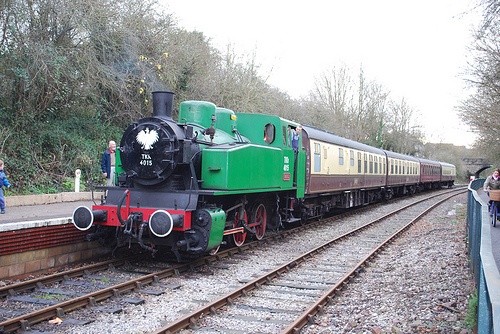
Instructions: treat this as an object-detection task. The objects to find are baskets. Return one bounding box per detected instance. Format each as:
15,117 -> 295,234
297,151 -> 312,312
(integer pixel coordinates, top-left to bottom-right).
489,190 -> 500,201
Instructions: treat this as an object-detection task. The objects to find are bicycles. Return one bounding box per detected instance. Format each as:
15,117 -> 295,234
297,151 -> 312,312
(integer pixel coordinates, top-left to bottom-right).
485,188 -> 500,227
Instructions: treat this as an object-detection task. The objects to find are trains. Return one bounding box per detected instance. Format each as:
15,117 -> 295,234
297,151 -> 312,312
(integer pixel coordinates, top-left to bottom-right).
73,90 -> 457,264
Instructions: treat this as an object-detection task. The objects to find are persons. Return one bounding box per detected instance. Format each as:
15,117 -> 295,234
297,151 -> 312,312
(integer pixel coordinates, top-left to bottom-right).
0,160 -> 11,214
101,140 -> 117,196
484,168 -> 500,221
